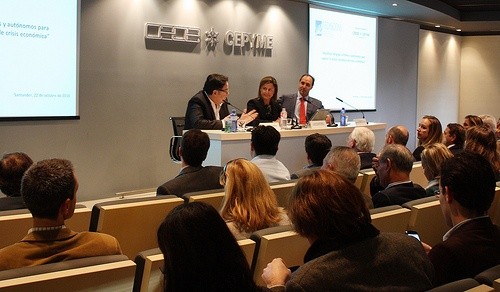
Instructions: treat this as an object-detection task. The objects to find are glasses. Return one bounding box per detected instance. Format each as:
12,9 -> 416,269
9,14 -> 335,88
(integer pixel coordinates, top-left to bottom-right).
221,88 -> 229,92
434,188 -> 444,199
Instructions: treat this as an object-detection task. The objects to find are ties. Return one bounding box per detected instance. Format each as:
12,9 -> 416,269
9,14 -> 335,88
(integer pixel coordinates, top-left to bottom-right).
300,98 -> 306,124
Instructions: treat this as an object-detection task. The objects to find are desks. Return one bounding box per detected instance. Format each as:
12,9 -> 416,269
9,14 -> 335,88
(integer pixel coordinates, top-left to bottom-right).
199,121 -> 387,175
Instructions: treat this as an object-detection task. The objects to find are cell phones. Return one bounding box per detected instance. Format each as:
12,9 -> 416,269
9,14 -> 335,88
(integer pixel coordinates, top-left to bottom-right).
406,230 -> 420,243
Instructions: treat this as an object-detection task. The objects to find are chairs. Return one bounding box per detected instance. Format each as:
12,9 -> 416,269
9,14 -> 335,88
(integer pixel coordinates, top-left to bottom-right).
169,116 -> 185,163
0,161 -> 500,292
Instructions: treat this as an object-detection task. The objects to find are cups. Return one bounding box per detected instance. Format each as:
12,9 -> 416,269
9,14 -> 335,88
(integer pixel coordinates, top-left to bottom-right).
237,120 -> 245,130
287,118 -> 292,130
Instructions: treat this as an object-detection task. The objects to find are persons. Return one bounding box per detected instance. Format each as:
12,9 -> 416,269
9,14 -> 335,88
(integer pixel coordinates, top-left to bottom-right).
250,125 -> 290,185
0,152 -> 34,197
292,114 -> 500,209
184,74 -> 258,130
0,159 -> 122,271
421,151 -> 500,288
262,169 -> 436,292
156,128 -> 224,198
156,202 -> 271,292
219,158 -> 290,241
247,76 -> 284,126
278,74 -> 334,124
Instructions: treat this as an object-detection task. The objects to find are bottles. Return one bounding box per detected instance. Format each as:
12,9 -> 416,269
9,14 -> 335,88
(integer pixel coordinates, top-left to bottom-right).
340,107 -> 347,126
280,107 -> 287,129
229,109 -> 238,131
326,115 -> 331,125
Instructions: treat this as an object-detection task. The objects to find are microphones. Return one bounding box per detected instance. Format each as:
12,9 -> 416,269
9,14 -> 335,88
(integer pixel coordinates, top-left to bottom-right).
274,101 -> 301,128
305,99 -> 330,125
222,99 -> 257,128
335,97 -> 366,123
